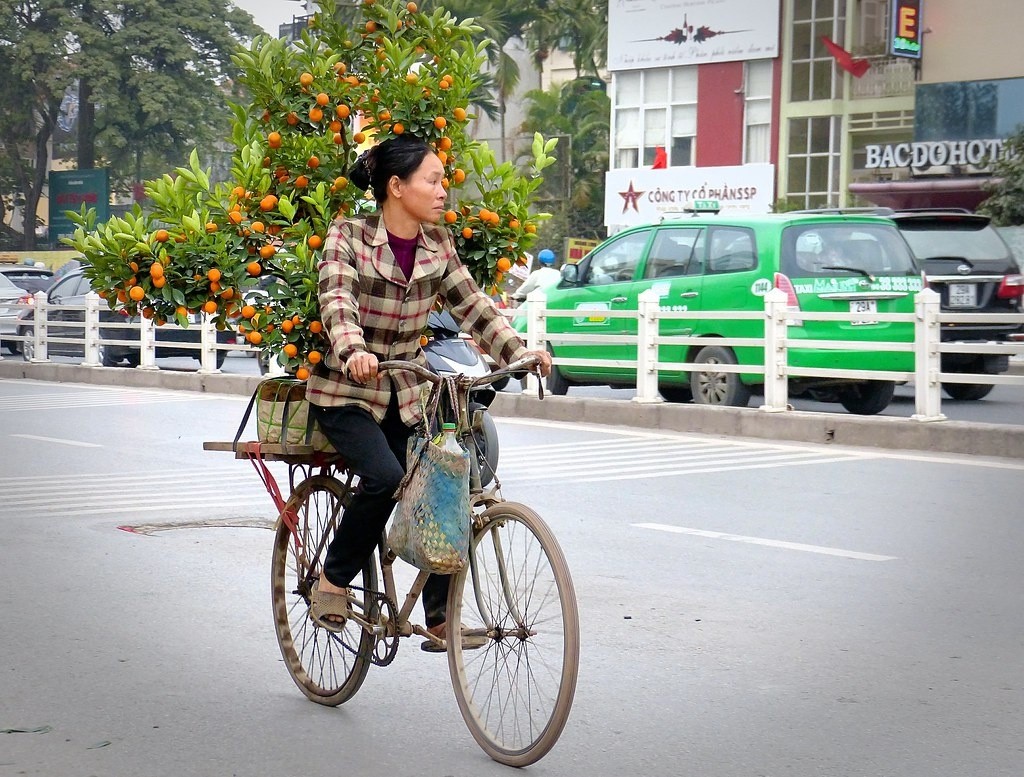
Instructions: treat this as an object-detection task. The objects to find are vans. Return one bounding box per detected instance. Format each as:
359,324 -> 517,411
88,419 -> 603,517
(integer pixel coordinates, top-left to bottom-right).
510,213 -> 927,416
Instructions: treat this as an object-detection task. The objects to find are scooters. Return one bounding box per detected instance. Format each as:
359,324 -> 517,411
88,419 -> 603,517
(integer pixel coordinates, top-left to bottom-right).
421,311 -> 500,490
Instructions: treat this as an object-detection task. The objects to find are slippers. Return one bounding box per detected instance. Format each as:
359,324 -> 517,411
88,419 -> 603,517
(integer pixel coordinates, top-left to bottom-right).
421,622 -> 489,652
312,580 -> 348,633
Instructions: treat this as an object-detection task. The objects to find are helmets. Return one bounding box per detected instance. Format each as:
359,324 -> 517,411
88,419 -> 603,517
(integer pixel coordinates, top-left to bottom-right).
538,250 -> 555,266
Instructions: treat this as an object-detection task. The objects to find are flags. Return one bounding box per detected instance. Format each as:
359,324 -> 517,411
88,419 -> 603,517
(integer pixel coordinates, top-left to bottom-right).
821,36 -> 872,78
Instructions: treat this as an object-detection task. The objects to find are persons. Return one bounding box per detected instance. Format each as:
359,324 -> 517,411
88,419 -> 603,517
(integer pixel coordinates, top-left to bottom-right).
646,238 -> 677,278
24,258 -> 46,269
306,134 -> 552,651
512,249 -> 562,322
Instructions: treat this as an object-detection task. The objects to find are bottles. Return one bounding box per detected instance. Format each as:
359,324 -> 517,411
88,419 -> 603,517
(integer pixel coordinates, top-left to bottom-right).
437,423 -> 465,459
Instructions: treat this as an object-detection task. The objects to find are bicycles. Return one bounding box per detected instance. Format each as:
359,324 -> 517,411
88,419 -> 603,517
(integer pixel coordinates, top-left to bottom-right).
271,356 -> 582,768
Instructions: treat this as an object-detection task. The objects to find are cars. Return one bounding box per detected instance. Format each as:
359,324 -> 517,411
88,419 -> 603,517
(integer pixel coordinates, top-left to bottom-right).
17,266 -> 235,371
0,262 -> 54,356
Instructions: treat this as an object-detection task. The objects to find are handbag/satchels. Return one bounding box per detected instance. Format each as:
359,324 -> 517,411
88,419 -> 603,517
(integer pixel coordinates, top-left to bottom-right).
386,374 -> 470,575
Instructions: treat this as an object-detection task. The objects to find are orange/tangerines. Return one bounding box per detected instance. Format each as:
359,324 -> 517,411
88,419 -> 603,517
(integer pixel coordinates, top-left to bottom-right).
93,0 -> 536,380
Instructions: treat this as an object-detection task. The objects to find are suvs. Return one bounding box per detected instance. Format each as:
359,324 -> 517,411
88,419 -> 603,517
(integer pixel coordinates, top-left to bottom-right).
732,206 -> 1024,400
